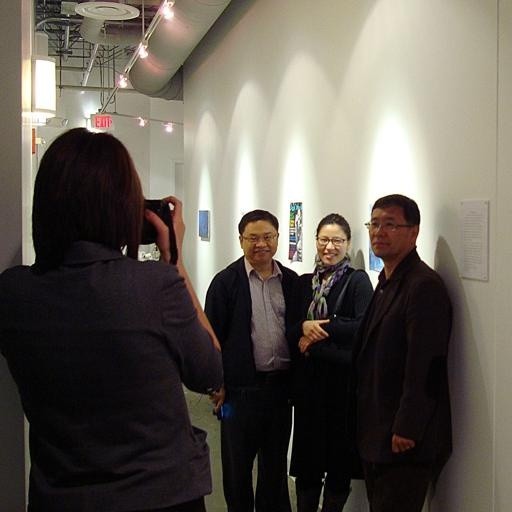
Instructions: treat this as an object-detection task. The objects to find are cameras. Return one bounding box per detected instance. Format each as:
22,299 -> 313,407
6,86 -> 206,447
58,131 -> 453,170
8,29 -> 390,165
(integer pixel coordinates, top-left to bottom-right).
140,199 -> 170,245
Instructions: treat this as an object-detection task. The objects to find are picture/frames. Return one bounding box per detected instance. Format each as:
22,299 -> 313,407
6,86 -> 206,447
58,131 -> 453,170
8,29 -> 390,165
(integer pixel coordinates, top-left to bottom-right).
198,210 -> 211,239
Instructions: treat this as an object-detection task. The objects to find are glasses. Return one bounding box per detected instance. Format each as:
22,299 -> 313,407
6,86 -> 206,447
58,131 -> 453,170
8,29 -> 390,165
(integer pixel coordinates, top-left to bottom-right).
244,234 -> 276,242
317,237 -> 346,246
365,222 -> 410,232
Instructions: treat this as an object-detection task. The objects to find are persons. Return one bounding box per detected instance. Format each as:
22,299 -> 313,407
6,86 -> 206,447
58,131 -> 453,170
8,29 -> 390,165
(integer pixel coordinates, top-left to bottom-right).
1,127 -> 222,511
202,210 -> 301,511
286,212 -> 375,512
347,194 -> 454,512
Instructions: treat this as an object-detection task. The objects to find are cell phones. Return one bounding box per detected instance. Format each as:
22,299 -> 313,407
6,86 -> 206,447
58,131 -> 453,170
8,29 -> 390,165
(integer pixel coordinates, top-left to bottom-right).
217,405 -> 223,421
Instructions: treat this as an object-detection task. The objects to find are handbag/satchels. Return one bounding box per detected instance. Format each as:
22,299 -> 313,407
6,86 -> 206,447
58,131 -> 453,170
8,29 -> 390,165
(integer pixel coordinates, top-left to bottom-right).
307,314 -> 357,359
